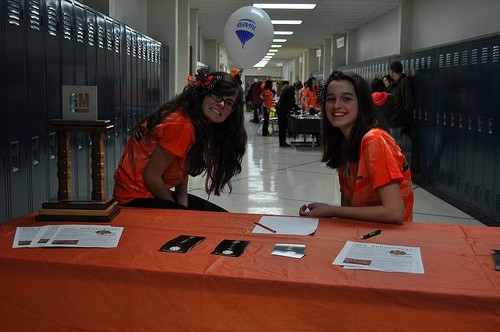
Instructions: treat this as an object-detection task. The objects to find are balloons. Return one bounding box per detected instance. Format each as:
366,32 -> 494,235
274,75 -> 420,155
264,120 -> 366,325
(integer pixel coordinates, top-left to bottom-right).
223,6 -> 274,70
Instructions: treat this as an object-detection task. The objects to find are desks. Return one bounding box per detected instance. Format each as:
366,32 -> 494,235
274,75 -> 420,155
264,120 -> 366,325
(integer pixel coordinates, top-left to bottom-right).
0,206 -> 499,332
291,113 -> 322,150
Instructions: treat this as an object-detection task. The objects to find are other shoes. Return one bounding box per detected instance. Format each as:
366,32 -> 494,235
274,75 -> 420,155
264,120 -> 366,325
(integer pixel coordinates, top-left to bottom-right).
280,143 -> 292,147
264,134 -> 272,136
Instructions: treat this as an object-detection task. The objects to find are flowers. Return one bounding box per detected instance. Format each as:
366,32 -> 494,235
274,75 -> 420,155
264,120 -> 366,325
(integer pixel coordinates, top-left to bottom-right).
187,66 -> 220,90
231,69 -> 243,86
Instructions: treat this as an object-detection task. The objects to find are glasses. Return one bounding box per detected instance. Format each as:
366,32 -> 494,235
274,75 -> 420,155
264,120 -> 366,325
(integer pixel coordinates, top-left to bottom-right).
209,91 -> 236,111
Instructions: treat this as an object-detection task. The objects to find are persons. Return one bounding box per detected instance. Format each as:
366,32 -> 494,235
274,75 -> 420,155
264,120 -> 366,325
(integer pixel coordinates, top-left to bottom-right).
371,61 -> 413,167
113,66 -> 247,213
275,80 -> 304,147
294,77 -> 326,115
298,70 -> 414,225
244,79 -> 289,136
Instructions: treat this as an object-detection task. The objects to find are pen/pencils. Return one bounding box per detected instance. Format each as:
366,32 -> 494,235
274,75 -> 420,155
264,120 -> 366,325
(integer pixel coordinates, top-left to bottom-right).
254,222 -> 276,233
362,230 -> 381,239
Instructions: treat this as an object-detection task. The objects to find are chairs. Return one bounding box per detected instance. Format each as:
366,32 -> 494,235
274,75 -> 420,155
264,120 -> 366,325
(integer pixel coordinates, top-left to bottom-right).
272,118 -> 280,133
256,109 -> 273,135
